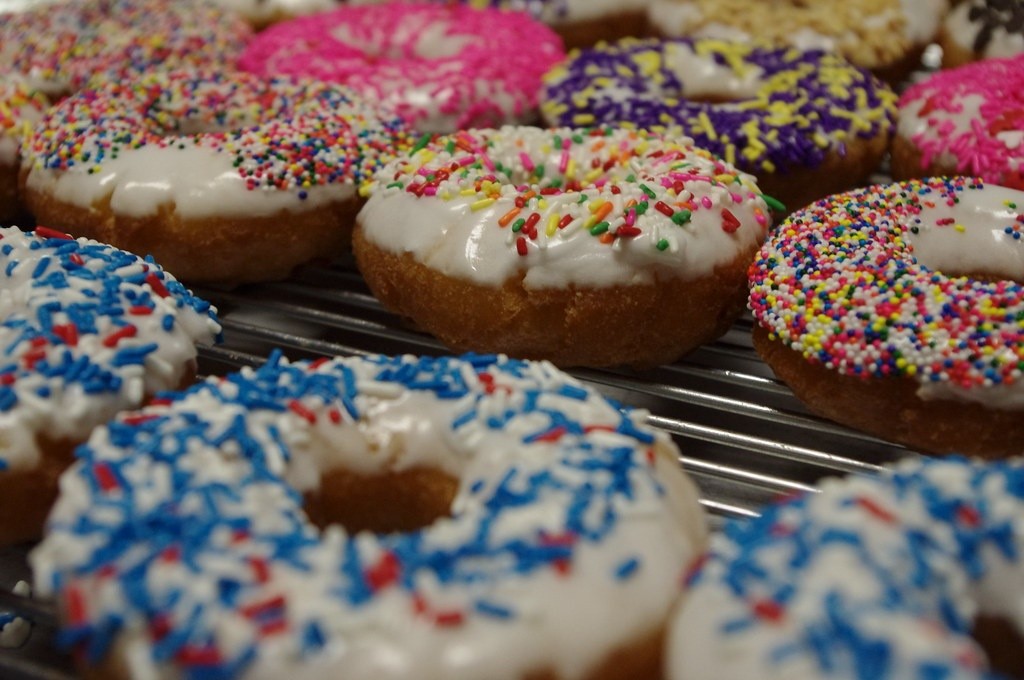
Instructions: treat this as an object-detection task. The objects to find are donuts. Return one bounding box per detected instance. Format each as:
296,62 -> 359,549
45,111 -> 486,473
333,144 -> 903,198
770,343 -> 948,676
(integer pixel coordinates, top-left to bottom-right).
0,0 -> 1024,680
